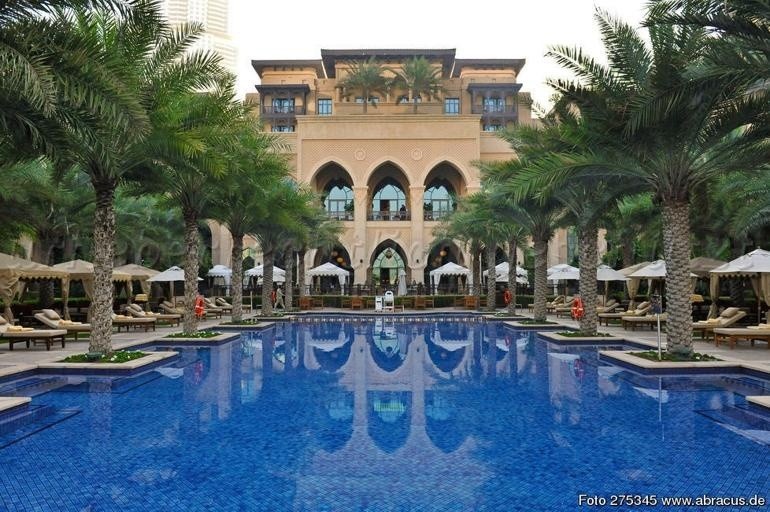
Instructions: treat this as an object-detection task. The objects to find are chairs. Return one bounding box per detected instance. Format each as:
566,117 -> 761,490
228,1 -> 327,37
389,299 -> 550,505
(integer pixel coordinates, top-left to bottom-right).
295,294 -> 489,311
527,294 -> 770,352
0,296 -> 254,352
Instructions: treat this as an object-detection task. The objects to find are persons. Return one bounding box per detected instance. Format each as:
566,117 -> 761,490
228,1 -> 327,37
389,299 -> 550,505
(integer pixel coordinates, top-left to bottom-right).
273,285 -> 286,310
423,202 -> 434,221
400,203 -> 407,220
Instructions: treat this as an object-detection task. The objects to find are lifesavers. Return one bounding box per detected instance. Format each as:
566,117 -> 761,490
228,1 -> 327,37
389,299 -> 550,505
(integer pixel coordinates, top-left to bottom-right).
504,291 -> 510,303
572,298 -> 583,318
272,291 -> 276,301
195,297 -> 205,314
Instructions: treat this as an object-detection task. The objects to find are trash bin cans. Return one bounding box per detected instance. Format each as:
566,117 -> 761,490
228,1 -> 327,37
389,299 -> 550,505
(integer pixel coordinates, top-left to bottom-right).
375,296 -> 382,312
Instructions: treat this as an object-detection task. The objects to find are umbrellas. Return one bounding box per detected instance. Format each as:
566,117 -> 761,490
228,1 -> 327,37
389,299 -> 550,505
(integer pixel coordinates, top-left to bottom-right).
716,420 -> 770,448
545,263 -> 576,275
708,245 -> 770,325
145,265 -> 205,309
397,323 -> 409,361
398,269 -> 408,297
628,259 -> 699,313
596,264 -> 632,307
548,267 -> 579,302
546,350 -> 671,403
154,364 -> 185,379
